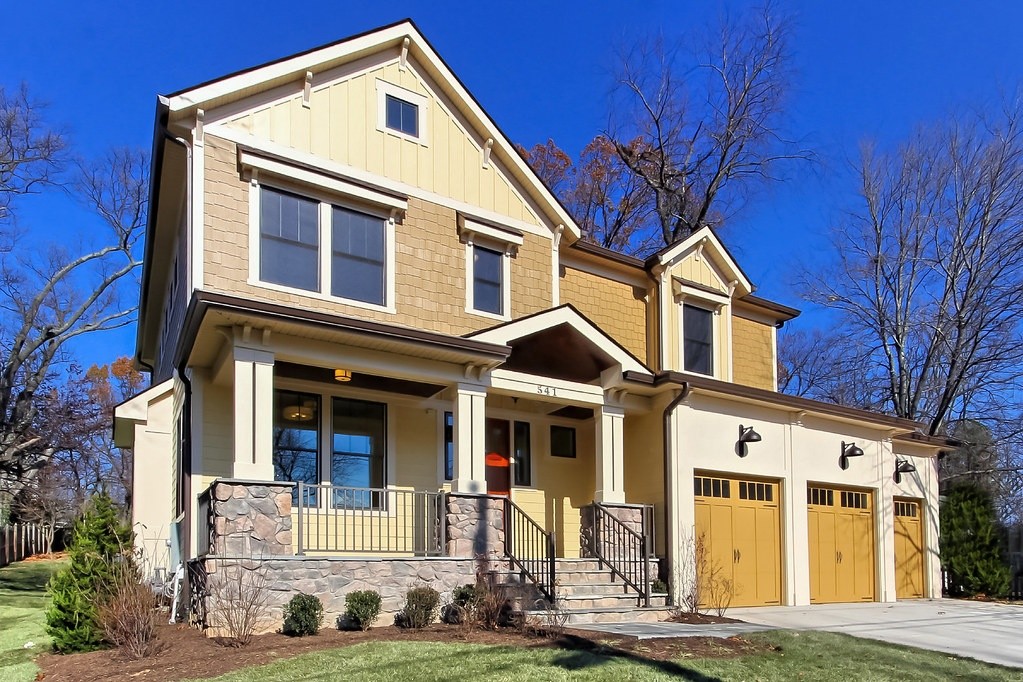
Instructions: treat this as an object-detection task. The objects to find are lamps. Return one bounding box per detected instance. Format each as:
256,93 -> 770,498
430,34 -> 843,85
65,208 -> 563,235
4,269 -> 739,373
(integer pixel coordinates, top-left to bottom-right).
895,457 -> 915,482
841,439 -> 864,466
334,369 -> 352,382
739,423 -> 764,455
283,405 -> 314,422
